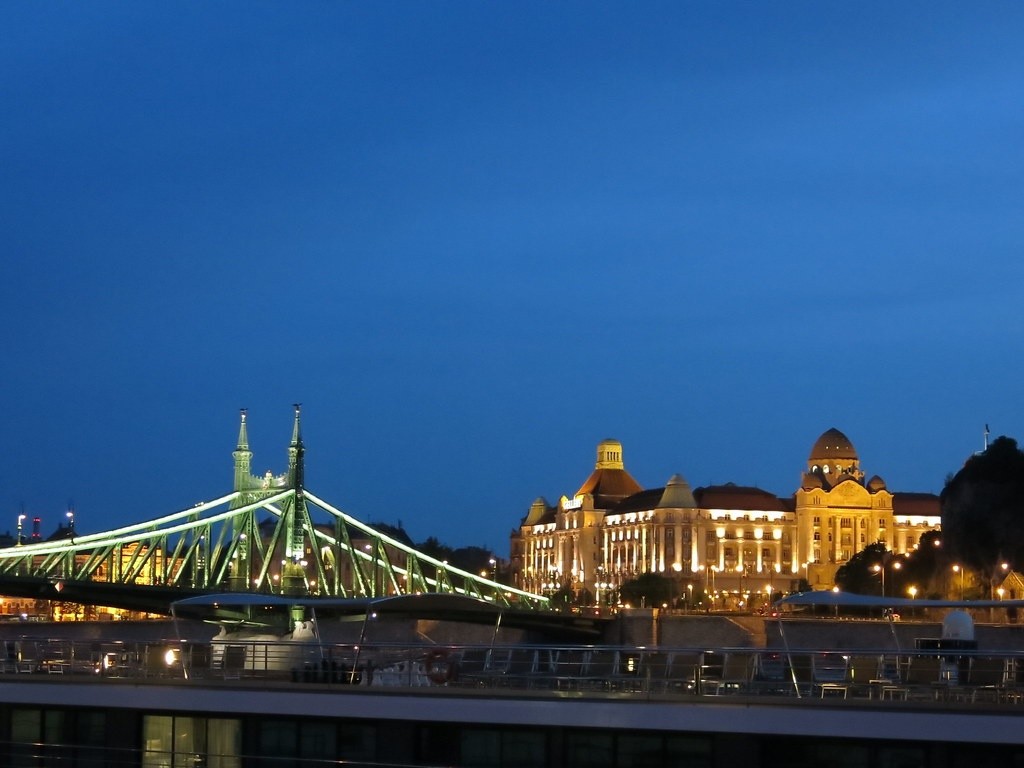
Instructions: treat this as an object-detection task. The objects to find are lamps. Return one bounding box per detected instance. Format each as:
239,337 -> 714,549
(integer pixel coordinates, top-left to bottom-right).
297,558 -> 309,567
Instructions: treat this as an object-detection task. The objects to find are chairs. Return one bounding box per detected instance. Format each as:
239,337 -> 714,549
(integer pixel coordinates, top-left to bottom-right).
0,638 -> 1023,708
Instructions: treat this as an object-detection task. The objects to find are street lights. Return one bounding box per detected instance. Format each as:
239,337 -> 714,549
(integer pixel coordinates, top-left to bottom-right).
736,566 -> 743,611
908,586 -> 917,620
833,585 -> 839,618
490,560 -> 497,583
874,565 -> 884,596
952,565 -> 964,601
